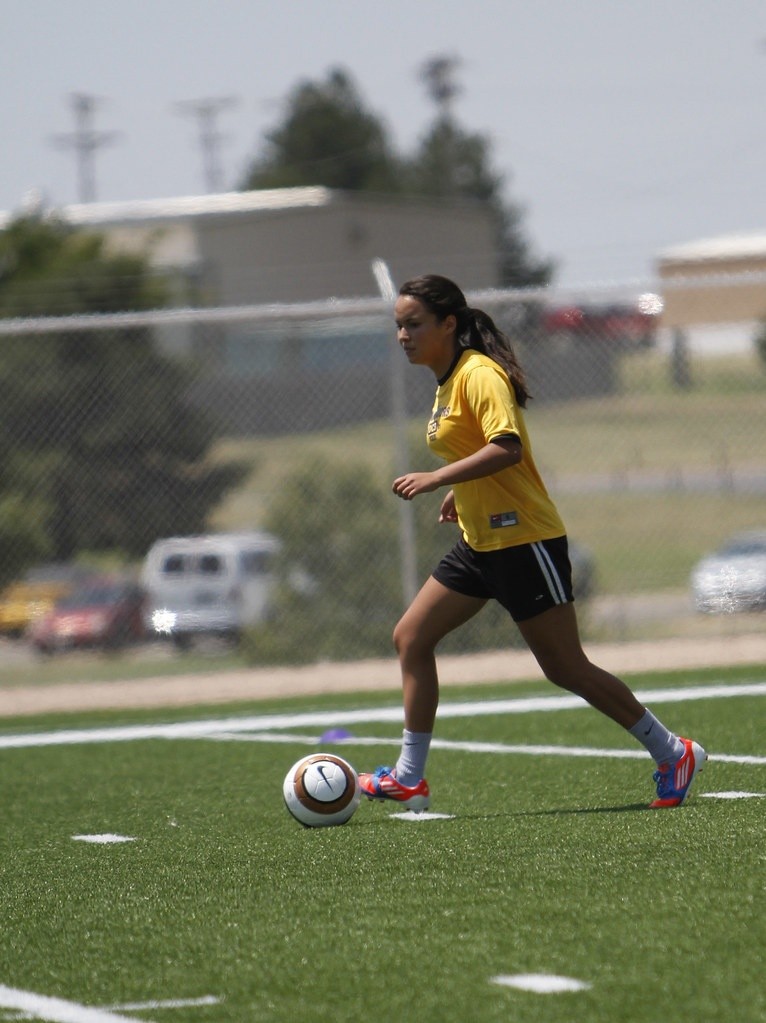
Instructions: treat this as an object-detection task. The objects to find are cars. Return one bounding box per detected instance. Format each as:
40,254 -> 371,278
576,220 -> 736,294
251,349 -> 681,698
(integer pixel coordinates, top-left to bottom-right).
0,558 -> 155,654
686,531 -> 766,612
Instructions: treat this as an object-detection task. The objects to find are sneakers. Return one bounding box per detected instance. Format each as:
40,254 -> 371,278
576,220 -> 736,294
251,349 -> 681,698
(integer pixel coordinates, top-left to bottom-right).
359,767 -> 430,815
651,738 -> 707,809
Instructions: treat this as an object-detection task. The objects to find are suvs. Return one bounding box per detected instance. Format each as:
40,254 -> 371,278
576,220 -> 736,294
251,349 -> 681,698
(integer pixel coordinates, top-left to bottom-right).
137,528 -> 316,649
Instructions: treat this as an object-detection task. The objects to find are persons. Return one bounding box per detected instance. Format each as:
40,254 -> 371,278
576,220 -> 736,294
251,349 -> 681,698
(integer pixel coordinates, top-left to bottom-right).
357,272 -> 708,813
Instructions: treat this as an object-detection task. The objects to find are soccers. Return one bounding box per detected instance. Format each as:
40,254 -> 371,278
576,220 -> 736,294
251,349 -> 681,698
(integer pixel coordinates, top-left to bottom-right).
280,752 -> 362,829
